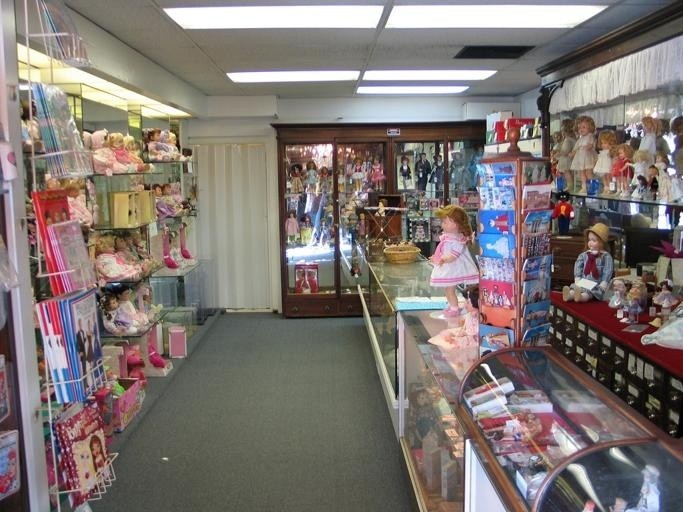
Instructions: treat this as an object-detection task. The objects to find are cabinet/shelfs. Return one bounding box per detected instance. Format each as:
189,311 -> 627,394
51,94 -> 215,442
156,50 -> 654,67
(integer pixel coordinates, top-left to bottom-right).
56,81 -> 167,339
358,248 -> 468,442
128,105 -> 201,378
455,346 -> 683,511
536,2 -> 683,442
399,309 -> 478,512
270,122 -> 391,318
392,122 -> 486,260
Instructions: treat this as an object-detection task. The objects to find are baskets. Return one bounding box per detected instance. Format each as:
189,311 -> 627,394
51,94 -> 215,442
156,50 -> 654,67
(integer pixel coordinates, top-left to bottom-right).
383,246 -> 421,264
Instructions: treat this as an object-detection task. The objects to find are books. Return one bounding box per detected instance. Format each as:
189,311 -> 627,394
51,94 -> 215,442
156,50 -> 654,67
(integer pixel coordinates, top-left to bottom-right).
29,83 -> 92,180
35,291 -> 108,402
55,396 -> 112,506
29,190 -> 94,296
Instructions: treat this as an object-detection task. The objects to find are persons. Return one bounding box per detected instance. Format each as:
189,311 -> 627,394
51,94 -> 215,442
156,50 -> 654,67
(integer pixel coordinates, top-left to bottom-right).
81,130 -> 164,335
562,223 -> 678,324
145,129 -> 191,268
428,206 -> 479,315
550,116 -> 683,201
399,154 -> 443,190
286,156 -> 384,245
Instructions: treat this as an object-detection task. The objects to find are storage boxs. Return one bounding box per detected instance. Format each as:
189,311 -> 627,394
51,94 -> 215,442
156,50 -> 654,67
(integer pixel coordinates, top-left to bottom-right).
108,377 -> 140,433
